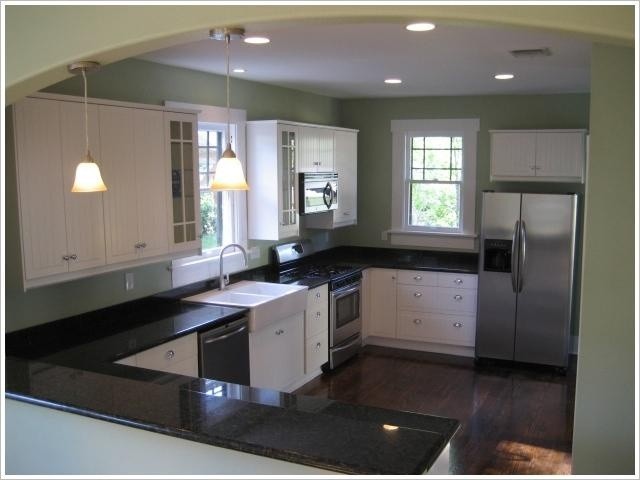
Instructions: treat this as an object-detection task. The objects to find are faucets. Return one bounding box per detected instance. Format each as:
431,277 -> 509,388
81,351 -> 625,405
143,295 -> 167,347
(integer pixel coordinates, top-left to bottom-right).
218,243 -> 248,290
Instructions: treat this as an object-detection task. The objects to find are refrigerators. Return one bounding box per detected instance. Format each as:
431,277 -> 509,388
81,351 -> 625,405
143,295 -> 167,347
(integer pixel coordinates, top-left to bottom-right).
474,188 -> 578,378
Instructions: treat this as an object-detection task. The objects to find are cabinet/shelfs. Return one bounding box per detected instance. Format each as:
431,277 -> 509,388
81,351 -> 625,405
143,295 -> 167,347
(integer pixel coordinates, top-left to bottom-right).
363,267 -> 398,346
305,284 -> 330,375
489,129 -> 587,183
297,122 -> 337,173
244,119 -> 299,241
168,110 -> 201,259
398,270 -> 479,357
114,332 -> 198,380
6,89 -> 105,292
249,310 -> 305,393
104,96 -> 173,272
334,127 -> 360,229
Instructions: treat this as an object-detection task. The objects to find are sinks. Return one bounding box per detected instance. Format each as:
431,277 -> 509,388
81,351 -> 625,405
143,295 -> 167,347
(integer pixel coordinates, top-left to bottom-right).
180,290 -> 275,309
226,279 -> 307,297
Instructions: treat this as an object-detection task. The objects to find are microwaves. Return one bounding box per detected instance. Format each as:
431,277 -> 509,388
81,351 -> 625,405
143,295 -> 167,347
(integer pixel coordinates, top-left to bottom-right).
298,171 -> 339,217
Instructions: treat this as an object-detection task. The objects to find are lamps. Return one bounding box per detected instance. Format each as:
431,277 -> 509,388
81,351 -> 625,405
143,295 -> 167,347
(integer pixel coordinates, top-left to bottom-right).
207,27 -> 250,191
68,61 -> 107,193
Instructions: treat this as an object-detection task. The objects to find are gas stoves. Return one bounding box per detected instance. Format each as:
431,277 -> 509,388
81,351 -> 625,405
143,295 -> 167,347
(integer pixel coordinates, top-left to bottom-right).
270,254 -> 369,290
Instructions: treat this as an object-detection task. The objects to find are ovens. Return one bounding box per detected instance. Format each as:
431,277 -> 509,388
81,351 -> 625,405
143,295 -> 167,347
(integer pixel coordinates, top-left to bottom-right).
328,281 -> 364,371
